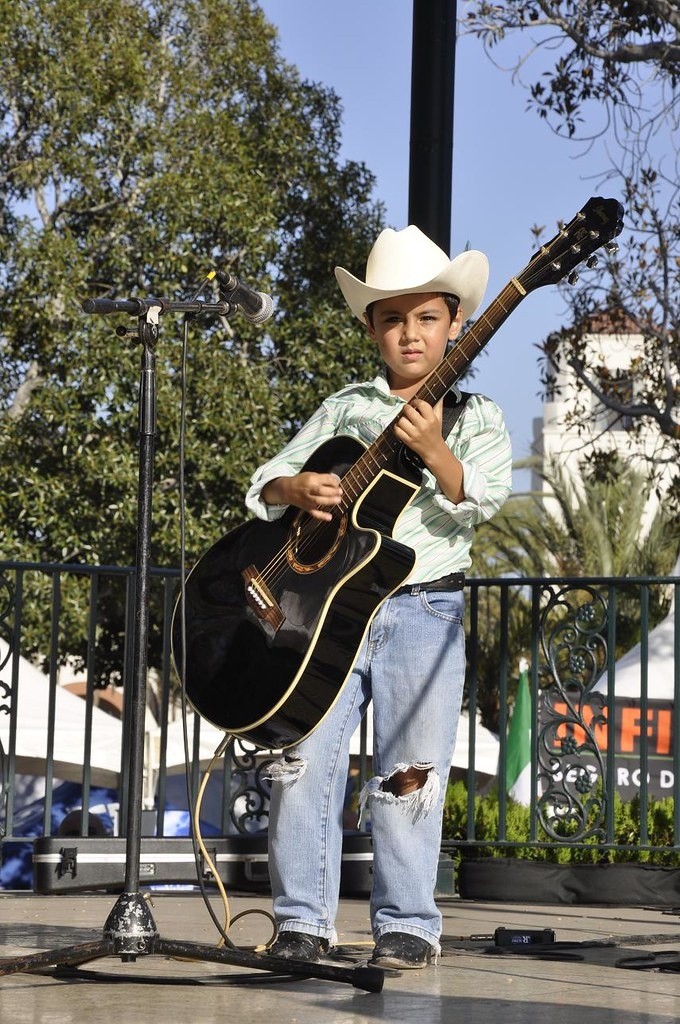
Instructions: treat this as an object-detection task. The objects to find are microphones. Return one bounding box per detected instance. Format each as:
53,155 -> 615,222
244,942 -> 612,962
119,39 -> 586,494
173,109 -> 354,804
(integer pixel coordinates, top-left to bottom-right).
211,266 -> 273,323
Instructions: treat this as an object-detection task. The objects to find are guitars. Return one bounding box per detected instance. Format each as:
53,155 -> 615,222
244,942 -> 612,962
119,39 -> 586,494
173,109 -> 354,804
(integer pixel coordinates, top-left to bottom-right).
167,197 -> 624,749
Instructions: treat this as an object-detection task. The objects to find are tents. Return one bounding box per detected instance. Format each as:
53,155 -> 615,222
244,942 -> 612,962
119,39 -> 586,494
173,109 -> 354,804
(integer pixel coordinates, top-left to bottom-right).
0,638 -> 541,834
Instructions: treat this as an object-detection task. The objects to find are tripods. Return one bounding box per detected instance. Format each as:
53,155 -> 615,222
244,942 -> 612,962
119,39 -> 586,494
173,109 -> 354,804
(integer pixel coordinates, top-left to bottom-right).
0,299 -> 385,993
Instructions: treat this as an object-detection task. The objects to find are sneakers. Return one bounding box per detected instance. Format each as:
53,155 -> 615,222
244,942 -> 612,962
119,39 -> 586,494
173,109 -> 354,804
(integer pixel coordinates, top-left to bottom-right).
269,931 -> 329,964
373,931 -> 432,968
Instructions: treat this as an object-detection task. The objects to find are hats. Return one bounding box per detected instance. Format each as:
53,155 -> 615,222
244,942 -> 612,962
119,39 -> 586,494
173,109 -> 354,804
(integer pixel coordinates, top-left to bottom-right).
335,225 -> 489,323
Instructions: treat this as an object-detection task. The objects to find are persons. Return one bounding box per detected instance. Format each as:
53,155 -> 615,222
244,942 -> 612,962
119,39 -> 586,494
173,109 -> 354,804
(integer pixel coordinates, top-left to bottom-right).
244,225 -> 511,966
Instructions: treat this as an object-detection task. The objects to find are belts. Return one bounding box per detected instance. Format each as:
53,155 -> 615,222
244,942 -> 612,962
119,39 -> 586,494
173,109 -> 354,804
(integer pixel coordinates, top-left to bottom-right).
386,572 -> 466,599
